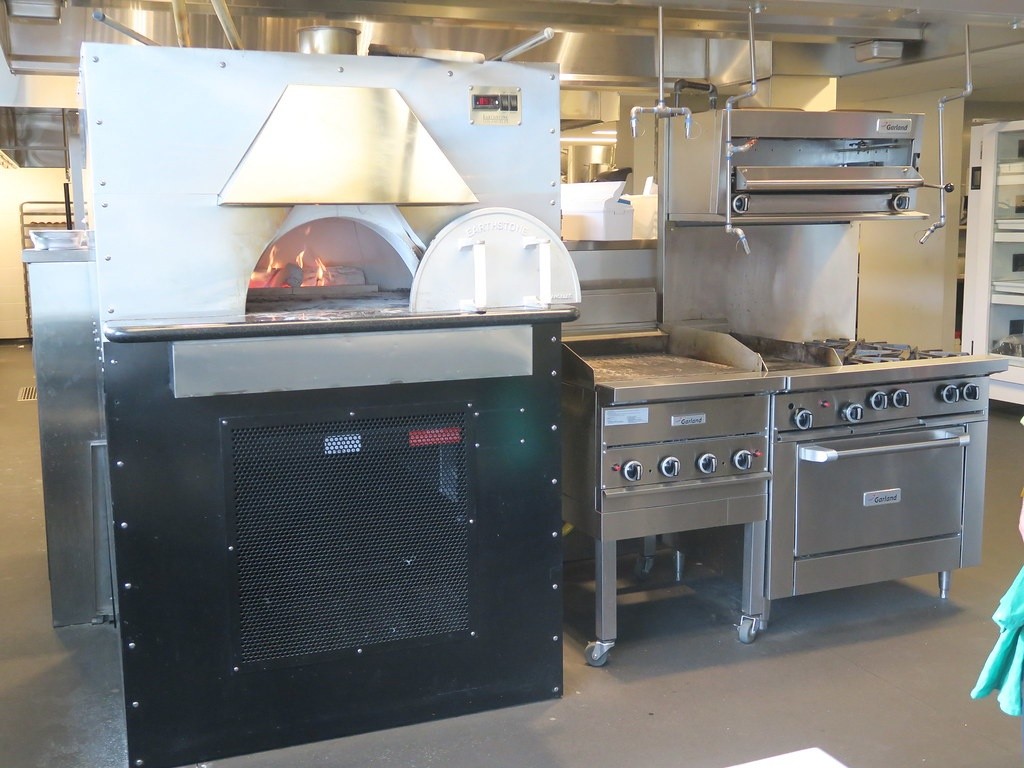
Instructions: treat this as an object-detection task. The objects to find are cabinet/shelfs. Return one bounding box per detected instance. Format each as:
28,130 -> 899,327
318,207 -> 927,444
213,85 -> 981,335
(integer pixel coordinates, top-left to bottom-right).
961,120 -> 1024,405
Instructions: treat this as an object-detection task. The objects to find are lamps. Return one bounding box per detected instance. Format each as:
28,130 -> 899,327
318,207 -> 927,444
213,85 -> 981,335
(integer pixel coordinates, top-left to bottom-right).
854,40 -> 904,64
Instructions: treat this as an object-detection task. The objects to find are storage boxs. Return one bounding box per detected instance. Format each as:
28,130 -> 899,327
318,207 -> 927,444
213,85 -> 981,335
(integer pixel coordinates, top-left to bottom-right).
561,181 -> 634,241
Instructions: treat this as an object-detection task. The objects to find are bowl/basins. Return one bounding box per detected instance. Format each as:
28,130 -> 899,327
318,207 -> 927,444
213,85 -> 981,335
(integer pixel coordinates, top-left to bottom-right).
27,230 -> 87,251
